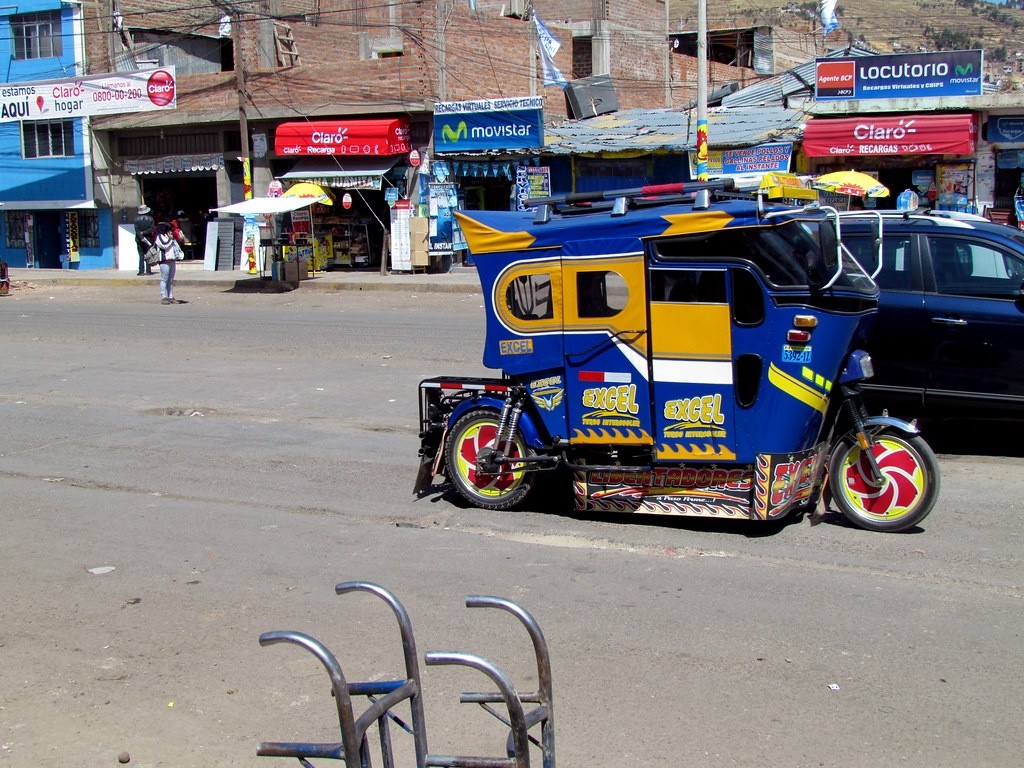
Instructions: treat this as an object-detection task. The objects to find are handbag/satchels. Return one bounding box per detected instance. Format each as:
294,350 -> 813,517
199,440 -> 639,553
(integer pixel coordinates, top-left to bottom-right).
178,230 -> 185,242
144,247 -> 158,265
171,240 -> 184,260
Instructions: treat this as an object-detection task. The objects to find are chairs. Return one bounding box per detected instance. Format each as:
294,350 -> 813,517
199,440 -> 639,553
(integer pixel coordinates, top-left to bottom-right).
932,241 -> 985,295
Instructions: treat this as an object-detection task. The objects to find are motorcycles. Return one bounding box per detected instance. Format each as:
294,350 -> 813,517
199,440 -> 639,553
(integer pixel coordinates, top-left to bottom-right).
412,171 -> 942,532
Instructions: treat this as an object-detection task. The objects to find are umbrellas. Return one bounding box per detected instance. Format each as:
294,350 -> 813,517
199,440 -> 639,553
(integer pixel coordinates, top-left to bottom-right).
812,168 -> 890,211
280,183 -> 336,271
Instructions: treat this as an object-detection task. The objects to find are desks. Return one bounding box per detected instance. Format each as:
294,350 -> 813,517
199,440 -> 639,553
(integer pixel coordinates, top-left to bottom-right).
260,244 -> 315,280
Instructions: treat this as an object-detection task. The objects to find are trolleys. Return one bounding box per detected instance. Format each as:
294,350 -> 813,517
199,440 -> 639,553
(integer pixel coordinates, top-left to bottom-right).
422,593 -> 556,767
258,581 -> 429,768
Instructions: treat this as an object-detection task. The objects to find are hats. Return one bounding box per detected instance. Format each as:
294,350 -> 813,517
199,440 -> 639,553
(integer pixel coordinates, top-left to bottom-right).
138,204 -> 151,214
178,211 -> 184,215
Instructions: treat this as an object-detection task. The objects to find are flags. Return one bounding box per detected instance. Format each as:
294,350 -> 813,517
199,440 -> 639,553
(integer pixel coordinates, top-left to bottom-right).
533,15 -> 565,85
818,0 -> 839,36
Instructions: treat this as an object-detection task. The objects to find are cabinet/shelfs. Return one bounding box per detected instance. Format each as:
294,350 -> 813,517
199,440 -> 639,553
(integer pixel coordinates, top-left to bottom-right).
281,223 -> 373,272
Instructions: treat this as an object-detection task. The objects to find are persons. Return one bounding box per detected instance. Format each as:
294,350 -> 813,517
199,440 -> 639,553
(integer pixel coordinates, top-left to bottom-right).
696,271 -> 752,319
178,211 -> 191,242
135,204 -> 155,276
851,200 -> 865,210
155,222 -> 179,304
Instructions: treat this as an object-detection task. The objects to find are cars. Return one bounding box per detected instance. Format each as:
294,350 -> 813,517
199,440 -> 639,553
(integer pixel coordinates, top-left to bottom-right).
830,208 -> 1023,457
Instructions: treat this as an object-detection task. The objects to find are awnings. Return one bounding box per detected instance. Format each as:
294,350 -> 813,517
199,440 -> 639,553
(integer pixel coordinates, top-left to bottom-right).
274,118 -> 412,156
803,112 -> 976,157
209,195 -> 321,279
274,156 -> 401,189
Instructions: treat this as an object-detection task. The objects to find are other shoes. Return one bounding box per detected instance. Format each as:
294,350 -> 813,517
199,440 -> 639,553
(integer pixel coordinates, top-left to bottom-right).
161,297 -> 175,304
147,272 -> 153,275
137,272 -> 144,276
